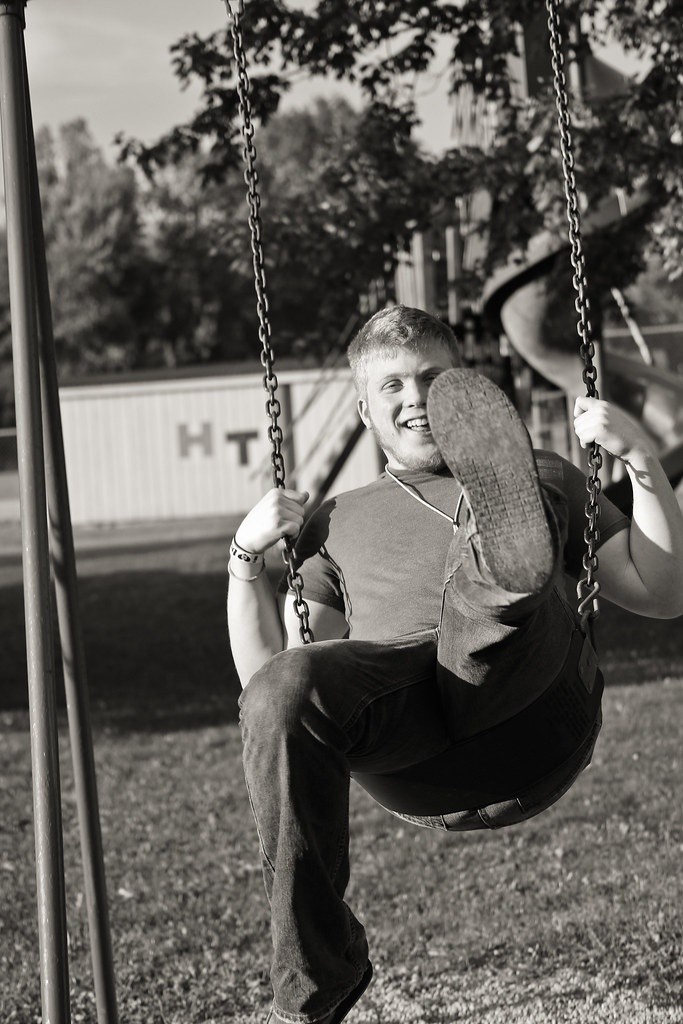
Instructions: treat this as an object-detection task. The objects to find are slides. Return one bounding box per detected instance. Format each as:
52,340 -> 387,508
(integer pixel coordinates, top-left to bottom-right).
474,172 -> 683,519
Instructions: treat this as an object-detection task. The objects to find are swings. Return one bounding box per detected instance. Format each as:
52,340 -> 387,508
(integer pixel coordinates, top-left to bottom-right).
214,0 -> 614,839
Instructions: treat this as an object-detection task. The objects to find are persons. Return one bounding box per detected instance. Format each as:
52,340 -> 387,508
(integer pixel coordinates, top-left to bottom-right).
227,305 -> 683,1020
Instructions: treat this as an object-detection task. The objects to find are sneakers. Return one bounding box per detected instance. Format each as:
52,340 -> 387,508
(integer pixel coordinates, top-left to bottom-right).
263,963 -> 375,1024
424,366 -> 556,593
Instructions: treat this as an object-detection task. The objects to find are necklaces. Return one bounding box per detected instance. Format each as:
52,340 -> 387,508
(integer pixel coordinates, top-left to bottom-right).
384,461 -> 464,536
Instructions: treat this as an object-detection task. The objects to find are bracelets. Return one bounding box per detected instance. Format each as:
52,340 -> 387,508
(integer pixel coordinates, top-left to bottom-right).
229,535 -> 264,565
228,558 -> 266,582
